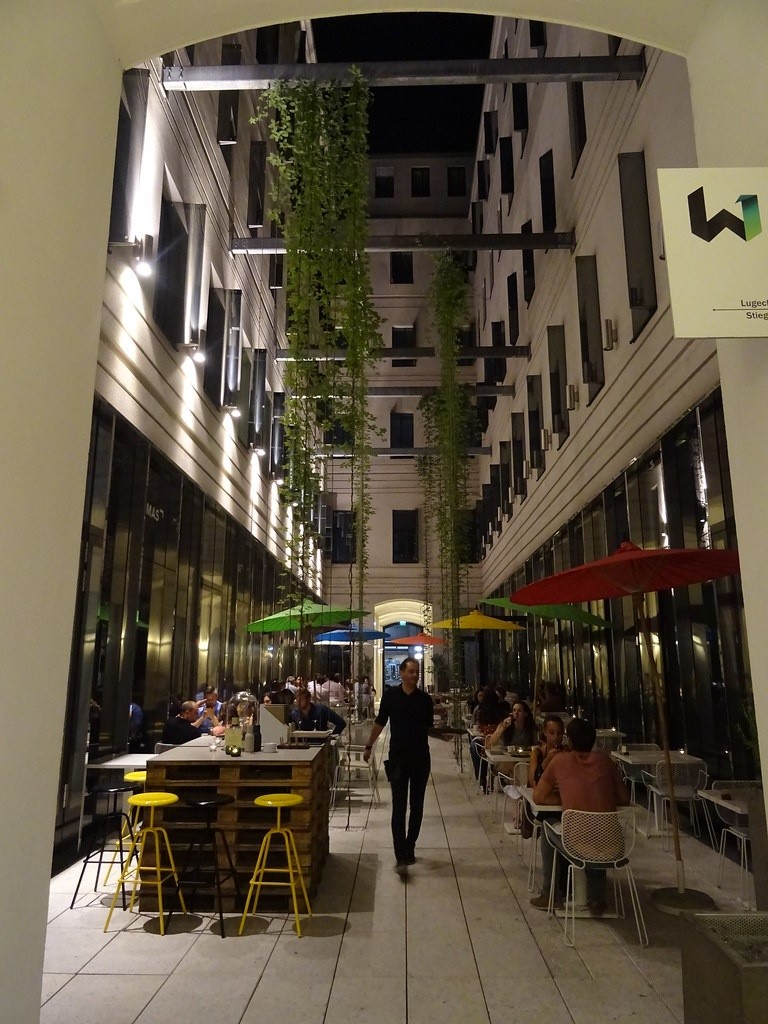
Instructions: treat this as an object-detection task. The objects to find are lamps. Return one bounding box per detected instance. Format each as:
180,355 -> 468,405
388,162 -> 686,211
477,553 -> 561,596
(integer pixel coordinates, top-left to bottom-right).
523,460 -> 532,479
132,234 -> 154,277
498,507 -> 505,521
602,319 -> 618,351
565,385 -> 579,410
509,487 -> 517,503
541,429 -> 551,450
191,330 -> 207,363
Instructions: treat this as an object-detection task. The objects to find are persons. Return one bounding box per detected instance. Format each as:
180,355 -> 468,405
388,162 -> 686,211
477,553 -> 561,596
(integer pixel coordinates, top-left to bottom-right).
286,689 -> 347,743
128,702 -> 143,753
363,657 -> 453,872
521,715 -> 630,916
264,672 -> 376,720
163,685 -> 239,745
467,684 -> 539,794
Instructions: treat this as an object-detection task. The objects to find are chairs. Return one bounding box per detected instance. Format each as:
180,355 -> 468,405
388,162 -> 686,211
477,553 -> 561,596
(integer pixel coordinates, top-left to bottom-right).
64,692 -> 381,938
460,712 -> 762,947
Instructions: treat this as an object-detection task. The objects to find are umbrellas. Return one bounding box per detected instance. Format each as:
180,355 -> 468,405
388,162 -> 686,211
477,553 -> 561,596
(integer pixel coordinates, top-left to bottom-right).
424,605 -> 526,691
478,598 -> 618,722
314,622 -> 391,705
390,630 -> 447,644
510,541 -> 740,890
241,598 -> 372,703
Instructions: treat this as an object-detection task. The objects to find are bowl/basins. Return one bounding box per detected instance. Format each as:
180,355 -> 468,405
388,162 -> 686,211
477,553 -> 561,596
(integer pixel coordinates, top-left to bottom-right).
507,746 -> 515,752
263,743 -> 277,753
531,746 -> 539,750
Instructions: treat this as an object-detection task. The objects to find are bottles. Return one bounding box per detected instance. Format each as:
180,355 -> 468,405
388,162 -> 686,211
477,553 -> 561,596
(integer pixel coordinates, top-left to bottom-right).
245,725 -> 254,752
254,725 -> 261,751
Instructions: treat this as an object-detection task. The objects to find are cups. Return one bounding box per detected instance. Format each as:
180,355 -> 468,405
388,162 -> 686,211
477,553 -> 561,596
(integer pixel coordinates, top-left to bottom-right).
209,742 -> 217,751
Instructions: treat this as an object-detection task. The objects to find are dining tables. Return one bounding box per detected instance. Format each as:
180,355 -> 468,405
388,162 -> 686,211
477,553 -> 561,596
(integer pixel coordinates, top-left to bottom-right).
85,753 -> 164,846
593,728 -> 627,754
609,750 -> 704,839
485,745 -> 534,836
694,787 -> 767,815
516,787 -> 640,920
466,728 -> 491,739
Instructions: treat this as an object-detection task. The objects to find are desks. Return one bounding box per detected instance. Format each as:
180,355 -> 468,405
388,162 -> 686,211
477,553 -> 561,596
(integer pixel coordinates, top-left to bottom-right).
136,734 -> 330,915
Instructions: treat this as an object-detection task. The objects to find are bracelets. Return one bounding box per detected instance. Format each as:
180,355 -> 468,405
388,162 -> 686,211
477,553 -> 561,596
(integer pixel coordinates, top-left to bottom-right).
364,745 -> 372,750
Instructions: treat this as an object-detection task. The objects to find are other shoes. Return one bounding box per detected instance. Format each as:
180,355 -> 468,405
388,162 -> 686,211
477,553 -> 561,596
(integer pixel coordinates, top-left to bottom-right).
530,890 -> 563,909
589,901 -> 606,916
406,840 -> 415,866
397,863 -> 408,876
